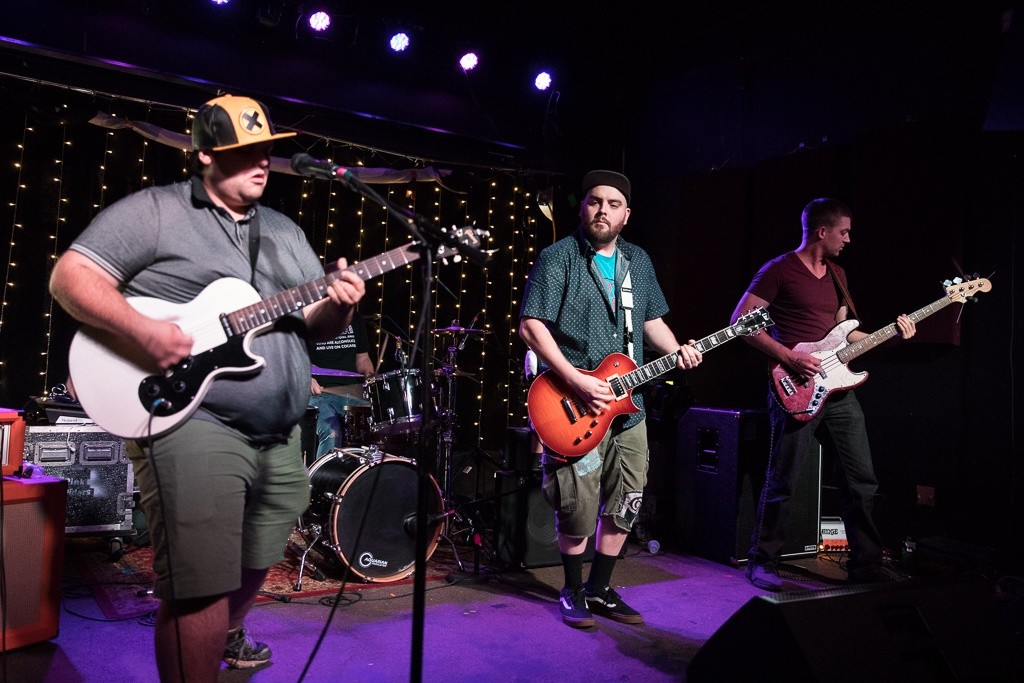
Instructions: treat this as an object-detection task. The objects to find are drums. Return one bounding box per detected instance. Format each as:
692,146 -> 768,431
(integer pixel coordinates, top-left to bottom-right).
346,405 -> 372,445
295,447 -> 446,583
361,367 -> 444,436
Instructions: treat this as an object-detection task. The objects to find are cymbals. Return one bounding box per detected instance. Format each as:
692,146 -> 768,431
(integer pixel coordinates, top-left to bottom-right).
431,368 -> 478,377
429,325 -> 491,335
311,364 -> 365,377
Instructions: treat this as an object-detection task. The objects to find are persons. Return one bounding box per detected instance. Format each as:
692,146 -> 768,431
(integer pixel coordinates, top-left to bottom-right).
45,94 -> 366,683
730,197 -> 917,592
305,260 -> 376,460
518,170 -> 704,628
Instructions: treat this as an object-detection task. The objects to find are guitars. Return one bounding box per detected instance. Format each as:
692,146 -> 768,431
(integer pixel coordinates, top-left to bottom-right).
770,274 -> 994,422
67,224 -> 494,438
525,303 -> 775,459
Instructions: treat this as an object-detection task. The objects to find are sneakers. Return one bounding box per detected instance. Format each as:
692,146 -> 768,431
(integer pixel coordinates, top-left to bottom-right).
847,558 -> 902,588
583,581 -> 642,623
223,627 -> 273,668
746,558 -> 785,591
559,587 -> 595,626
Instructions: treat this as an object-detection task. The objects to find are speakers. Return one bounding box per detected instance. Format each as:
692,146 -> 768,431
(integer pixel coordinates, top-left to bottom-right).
684,581 -> 949,683
671,407 -> 824,565
481,466 -> 628,568
0,476 -> 68,652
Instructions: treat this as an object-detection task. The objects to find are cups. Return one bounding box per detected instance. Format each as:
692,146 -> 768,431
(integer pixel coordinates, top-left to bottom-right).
648,540 -> 661,554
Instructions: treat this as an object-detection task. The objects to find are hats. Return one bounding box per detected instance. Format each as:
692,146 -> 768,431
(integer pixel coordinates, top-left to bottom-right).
191,94 -> 297,151
581,169 -> 632,208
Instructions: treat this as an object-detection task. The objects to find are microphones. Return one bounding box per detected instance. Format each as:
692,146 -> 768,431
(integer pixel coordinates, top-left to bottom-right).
363,313 -> 382,322
290,152 -> 352,180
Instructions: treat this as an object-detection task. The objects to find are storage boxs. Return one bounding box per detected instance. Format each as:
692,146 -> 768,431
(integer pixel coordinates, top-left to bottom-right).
23,424 -> 134,532
